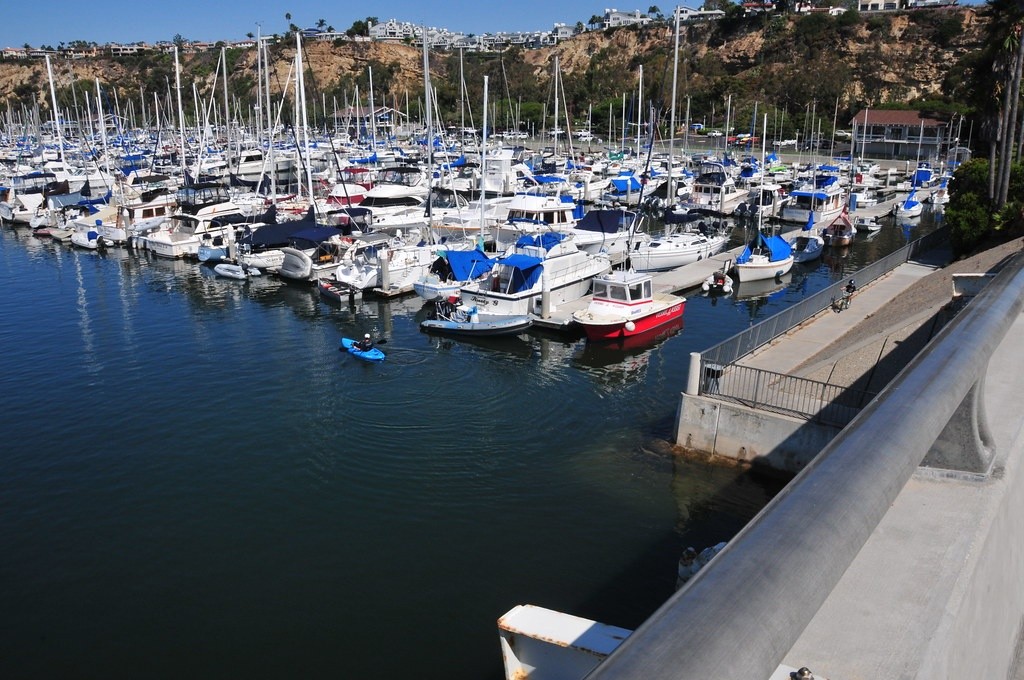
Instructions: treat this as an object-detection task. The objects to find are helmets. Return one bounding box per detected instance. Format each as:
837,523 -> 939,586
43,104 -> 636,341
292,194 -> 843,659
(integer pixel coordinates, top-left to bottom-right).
365,333 -> 371,339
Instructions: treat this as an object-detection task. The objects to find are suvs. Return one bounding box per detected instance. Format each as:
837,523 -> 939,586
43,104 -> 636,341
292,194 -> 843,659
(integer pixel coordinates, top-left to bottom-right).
835,130 -> 851,137
824,140 -> 837,148
806,140 -> 827,148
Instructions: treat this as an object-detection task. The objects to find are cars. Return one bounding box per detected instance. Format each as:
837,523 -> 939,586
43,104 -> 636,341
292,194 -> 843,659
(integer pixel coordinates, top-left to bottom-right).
490,131 -> 528,140
785,137 -> 796,145
728,133 -> 760,146
773,139 -> 786,146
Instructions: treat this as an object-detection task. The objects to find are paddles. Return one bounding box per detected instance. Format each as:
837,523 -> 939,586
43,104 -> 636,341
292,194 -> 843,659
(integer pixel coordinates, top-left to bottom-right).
339,339 -> 388,352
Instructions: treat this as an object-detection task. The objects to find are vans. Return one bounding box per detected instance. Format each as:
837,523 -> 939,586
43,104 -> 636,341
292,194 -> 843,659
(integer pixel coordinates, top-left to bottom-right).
689,124 -> 702,130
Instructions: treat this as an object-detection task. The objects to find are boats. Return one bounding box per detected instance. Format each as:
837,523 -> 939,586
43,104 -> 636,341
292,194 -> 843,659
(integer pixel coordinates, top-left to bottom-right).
572,267 -> 688,340
824,214 -> 856,248
214,264 -> 261,279
328,207 -> 445,289
95,199 -> 186,249
146,180 -> 268,259
275,239 -> 352,284
197,218 -> 268,264
858,216 -> 882,233
929,174 -> 950,205
701,271 -> 733,294
577,319 -> 684,393
420,230 -> 612,335
317,277 -> 363,301
342,337 -> 386,360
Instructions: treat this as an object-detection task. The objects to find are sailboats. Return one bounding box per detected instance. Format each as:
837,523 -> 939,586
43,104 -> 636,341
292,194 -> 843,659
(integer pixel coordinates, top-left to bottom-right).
373,21 -> 487,295
733,274 -> 793,330
415,5 -> 731,226
0,51 -> 99,251
228,34 -> 345,270
892,118 -> 923,218
733,100 -> 891,222
412,74 -> 513,296
100,41 -> 449,203
789,94 -> 826,261
897,216 -> 921,245
734,111 -> 795,283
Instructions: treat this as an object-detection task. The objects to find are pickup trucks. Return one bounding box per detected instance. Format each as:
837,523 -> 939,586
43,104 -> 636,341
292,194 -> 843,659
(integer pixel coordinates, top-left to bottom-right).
571,128 -> 590,138
548,128 -> 565,135
707,130 -> 722,138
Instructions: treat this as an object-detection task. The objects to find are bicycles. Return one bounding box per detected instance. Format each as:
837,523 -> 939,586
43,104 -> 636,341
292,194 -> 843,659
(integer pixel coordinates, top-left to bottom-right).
838,290 -> 853,312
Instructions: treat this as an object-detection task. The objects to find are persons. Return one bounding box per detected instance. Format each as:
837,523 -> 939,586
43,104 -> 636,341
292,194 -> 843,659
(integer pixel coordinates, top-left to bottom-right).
839,279 -> 856,309
242,263 -> 248,276
353,334 -> 374,352
